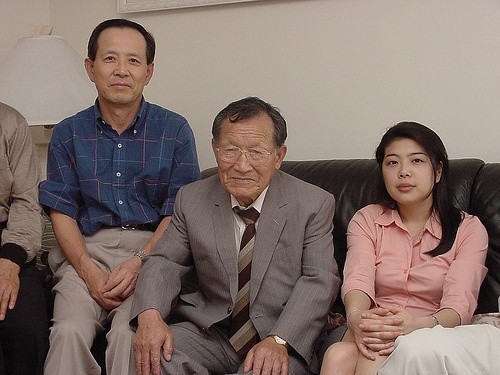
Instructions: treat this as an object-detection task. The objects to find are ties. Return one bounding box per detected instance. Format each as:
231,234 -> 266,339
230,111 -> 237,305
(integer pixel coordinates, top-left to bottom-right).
229,207 -> 260,357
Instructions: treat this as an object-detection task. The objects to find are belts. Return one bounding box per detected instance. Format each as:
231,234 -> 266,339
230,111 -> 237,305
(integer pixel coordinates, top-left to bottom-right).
119,223 -> 157,232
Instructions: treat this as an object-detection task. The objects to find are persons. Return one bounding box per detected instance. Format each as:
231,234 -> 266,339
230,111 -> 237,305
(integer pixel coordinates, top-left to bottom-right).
375,295 -> 500,375
0,102 -> 54,375
37,18 -> 202,375
318,119 -> 491,374
125,95 -> 342,374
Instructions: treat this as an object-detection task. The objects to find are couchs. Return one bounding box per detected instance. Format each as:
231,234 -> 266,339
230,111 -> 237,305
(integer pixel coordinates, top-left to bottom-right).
199,158 -> 500,316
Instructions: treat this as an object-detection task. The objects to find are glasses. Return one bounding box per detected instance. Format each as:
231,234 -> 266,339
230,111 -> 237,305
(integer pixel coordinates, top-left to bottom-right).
214,140 -> 279,161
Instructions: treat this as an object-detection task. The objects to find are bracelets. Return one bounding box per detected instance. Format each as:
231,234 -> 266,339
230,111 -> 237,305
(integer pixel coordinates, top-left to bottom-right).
431,315 -> 440,328
134,250 -> 149,262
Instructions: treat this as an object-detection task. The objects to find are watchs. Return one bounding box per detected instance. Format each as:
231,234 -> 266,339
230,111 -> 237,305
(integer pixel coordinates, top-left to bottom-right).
271,334 -> 288,349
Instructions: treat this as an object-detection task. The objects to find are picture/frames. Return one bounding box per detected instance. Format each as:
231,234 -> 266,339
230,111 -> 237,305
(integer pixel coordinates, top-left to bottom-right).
117,0 -> 259,14
